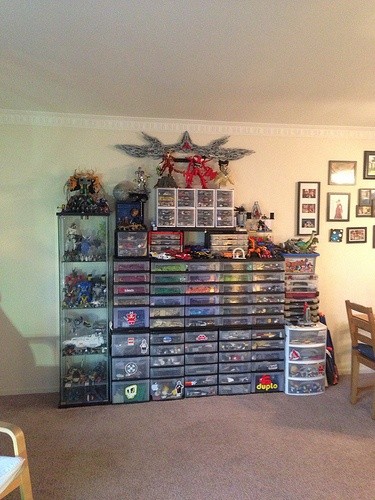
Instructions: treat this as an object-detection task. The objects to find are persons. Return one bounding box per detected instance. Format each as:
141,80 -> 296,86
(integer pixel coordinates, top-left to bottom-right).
65,223 -> 76,250
302,219 -> 315,228
159,151 -> 175,176
363,192 -> 375,205
302,189 -> 316,198
350,231 -> 365,240
185,154 -> 217,189
213,159 -> 236,188
334,200 -> 343,218
302,204 -> 315,213
331,230 -> 340,242
357,207 -> 370,215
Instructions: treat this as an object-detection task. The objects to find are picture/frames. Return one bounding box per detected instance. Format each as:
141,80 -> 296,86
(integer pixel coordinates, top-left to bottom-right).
346,227 -> 367,243
298,181 -> 321,235
363,151 -> 375,179
325,192 -> 351,222
327,160 -> 357,186
329,229 -> 343,242
356,188 -> 375,217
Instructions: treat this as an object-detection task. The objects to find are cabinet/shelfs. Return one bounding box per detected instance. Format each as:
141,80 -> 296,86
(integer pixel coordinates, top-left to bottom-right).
57,189 -> 329,407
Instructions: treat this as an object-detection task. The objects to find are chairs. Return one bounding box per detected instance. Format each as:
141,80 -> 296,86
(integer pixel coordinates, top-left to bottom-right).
0,420 -> 33,500
344,300 -> 375,404
